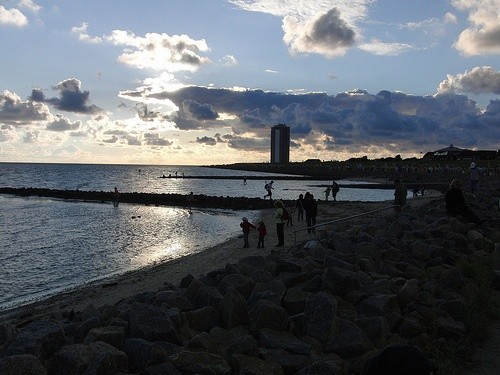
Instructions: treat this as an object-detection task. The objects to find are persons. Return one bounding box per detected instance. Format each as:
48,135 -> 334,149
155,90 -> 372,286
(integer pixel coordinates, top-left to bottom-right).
468,162 -> 480,198
264,181 -> 274,200
324,181 -> 339,200
447,180 -> 484,229
274,200 -> 286,247
258,222 -> 266,248
393,179 -> 407,215
186,191 -> 194,211
413,184 -> 425,198
239,217 -> 256,248
287,205 -> 295,227
297,194 -> 305,221
303,192 -> 318,233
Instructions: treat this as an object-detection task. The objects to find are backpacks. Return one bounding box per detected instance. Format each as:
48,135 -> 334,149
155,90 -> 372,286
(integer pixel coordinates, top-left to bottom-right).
265,184 -> 268,189
280,207 -> 288,220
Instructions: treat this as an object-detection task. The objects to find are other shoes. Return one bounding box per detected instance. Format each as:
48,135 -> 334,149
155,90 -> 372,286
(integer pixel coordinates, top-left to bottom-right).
276,244 -> 282,247
264,196 -> 266,199
261,246 -> 264,248
257,246 -> 259,248
270,199 -> 273,200
